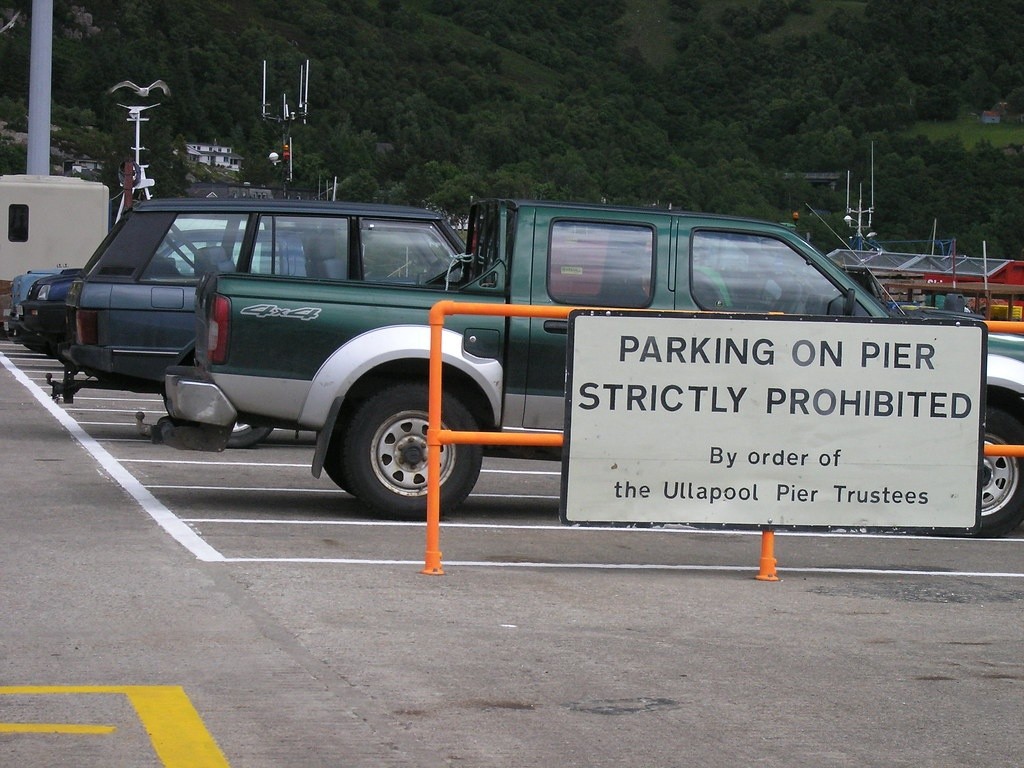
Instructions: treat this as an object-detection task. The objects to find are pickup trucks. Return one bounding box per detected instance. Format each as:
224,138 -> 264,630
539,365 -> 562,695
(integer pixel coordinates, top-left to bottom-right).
132,198 -> 1023,535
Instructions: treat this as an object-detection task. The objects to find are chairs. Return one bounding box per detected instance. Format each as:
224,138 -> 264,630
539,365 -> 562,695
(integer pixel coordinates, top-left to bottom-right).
193,245 -> 237,275
692,265 -> 732,306
303,235 -> 347,280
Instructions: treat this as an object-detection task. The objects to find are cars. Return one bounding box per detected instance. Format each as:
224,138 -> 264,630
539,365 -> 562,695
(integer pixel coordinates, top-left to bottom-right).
5,268 -> 82,358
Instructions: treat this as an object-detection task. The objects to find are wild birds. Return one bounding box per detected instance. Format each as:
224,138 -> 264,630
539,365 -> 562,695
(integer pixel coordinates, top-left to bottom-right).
104,79 -> 170,97
0,11 -> 21,33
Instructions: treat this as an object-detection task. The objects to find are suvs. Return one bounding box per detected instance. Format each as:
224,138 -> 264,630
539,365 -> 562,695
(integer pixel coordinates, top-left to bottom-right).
47,195 -> 470,448
